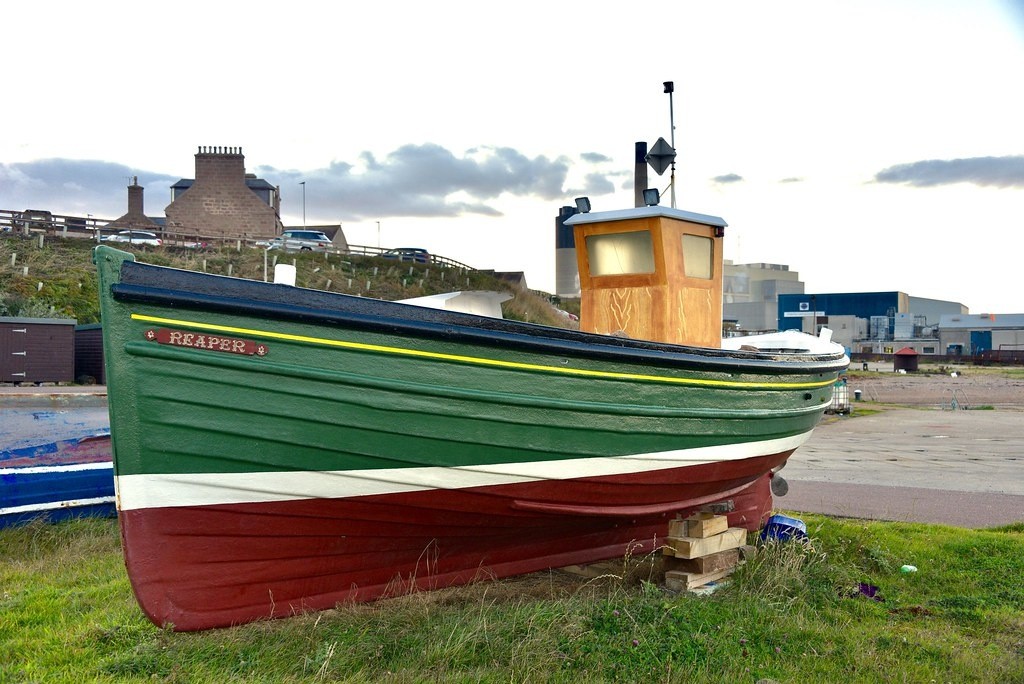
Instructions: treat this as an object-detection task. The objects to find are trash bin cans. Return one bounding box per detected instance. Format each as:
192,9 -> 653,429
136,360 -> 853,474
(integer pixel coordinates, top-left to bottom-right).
863,363 -> 868,371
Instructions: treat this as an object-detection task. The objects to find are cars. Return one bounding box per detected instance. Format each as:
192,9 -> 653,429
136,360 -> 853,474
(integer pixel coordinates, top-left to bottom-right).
90,231 -> 163,246
255,230 -> 335,253
374,247 -> 431,263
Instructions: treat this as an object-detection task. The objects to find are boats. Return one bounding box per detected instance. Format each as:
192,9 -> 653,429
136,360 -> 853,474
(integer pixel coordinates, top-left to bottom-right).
94,82 -> 851,640
0,386 -> 117,528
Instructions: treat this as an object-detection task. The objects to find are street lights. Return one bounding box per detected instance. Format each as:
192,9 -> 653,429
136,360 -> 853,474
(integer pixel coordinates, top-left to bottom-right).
376,222 -> 380,247
87,214 -> 93,229
299,181 -> 305,231
